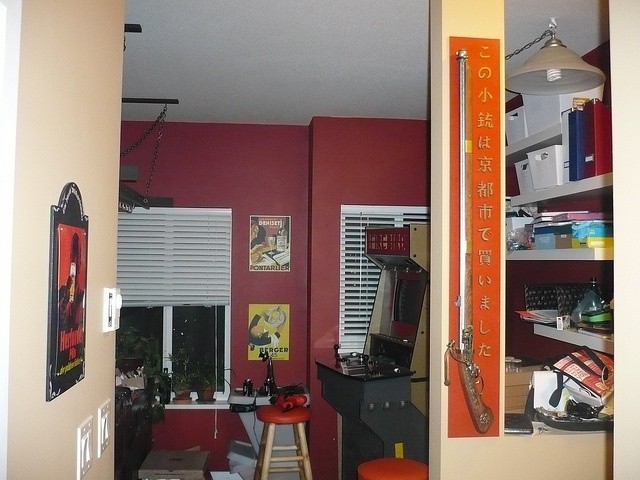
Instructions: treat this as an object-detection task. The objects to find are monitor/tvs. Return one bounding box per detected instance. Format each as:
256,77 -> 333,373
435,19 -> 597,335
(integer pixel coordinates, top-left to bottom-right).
392,272 -> 426,326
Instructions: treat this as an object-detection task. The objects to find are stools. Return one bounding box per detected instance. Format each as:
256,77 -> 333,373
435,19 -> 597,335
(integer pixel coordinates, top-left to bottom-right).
254,405 -> 314,480
357,456 -> 429,480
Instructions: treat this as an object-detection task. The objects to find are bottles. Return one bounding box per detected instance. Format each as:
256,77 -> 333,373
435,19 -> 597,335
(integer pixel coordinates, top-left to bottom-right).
512,359 -> 523,373
161,367 -> 172,404
505,355 -> 514,371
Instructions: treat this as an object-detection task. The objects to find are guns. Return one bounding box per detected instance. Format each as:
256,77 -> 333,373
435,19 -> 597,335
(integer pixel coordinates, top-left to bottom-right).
443,49 -> 494,434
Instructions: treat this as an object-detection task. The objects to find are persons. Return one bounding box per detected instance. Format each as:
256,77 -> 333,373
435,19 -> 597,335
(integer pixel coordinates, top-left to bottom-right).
58,231 -> 86,332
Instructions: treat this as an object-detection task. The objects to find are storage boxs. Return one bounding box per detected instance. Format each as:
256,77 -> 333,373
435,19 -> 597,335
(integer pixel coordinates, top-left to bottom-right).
514,158 -> 534,194
505,106 -> 529,145
527,145 -> 564,190
554,224 -> 572,249
521,83 -> 605,135
534,228 -> 555,249
138,451 -> 210,480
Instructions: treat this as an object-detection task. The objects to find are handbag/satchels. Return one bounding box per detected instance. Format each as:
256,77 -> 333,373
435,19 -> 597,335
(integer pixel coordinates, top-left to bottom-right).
563,365 -> 614,408
553,345 -> 614,398
532,368 -> 569,412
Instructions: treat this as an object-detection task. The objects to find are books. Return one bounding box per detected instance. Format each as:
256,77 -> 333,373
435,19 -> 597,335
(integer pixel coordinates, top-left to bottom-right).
534,211 -> 610,229
500,92 -> 612,248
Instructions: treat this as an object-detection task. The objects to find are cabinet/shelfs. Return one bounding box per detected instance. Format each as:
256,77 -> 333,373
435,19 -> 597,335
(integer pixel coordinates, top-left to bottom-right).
506,121 -> 614,355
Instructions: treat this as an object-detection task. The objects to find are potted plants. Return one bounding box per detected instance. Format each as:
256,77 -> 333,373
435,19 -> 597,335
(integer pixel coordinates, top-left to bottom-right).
194,364 -> 236,402
169,371 -> 193,404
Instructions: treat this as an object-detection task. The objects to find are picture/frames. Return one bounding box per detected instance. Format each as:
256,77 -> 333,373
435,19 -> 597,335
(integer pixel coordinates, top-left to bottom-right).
249,213 -> 293,274
46,181 -> 89,402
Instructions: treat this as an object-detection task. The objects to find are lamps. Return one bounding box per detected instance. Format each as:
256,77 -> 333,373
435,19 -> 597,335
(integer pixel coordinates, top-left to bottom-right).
505,17 -> 606,96
